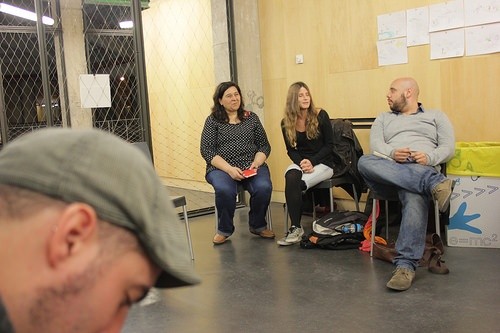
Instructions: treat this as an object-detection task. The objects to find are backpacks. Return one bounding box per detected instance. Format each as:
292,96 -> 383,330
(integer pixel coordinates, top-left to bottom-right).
299,209 -> 368,250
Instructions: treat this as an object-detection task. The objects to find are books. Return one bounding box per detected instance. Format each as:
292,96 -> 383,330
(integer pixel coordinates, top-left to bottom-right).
242,168 -> 257,178
373,149 -> 395,161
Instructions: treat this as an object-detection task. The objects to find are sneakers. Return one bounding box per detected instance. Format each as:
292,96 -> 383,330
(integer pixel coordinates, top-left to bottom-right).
277,232 -> 293,245
286,225 -> 304,243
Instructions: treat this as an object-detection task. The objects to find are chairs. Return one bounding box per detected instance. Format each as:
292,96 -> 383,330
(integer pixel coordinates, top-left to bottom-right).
131,141 -> 195,260
368,162 -> 446,257
284,119 -> 359,237
214,181 -> 273,233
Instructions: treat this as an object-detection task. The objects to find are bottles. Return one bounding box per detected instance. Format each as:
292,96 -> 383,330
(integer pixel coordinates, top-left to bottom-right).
343,224 -> 364,233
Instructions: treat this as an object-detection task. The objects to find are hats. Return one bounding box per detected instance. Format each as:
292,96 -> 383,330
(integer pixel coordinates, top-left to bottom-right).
1,126 -> 202,290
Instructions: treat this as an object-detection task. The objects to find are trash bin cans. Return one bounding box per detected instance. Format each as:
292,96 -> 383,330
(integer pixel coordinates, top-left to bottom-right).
445,142 -> 500,249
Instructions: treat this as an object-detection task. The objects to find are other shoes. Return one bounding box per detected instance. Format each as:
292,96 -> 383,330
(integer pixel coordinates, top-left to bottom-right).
386,265 -> 416,290
433,179 -> 455,212
256,228 -> 277,239
213,232 -> 229,244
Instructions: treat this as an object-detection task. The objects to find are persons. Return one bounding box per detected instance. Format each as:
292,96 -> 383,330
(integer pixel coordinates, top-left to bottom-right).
0,126 -> 201,333
277,82 -> 336,246
199,82 -> 275,243
357,77 -> 456,292
